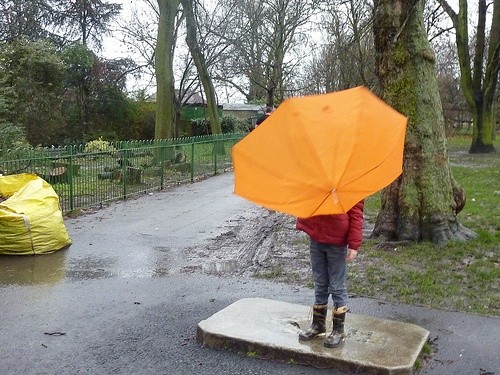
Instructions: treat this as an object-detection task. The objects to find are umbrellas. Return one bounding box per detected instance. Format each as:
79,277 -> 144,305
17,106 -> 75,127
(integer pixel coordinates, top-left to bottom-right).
232,85 -> 407,218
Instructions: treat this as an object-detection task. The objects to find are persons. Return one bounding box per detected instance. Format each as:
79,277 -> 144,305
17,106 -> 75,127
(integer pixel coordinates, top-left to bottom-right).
294,194 -> 365,347
255,106 -> 274,129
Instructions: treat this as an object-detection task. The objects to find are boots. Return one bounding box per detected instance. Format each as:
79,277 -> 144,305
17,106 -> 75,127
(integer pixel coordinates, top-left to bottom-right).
324,307 -> 346,348
298,305 -> 329,342
